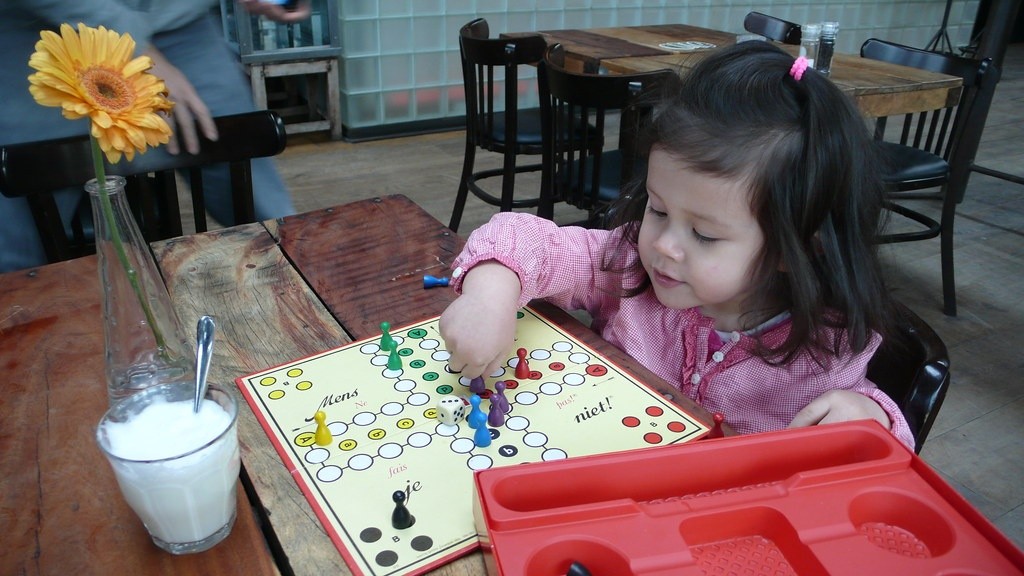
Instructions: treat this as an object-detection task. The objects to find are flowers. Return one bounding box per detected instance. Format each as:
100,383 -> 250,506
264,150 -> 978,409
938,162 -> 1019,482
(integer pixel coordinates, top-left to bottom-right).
27,22 -> 175,371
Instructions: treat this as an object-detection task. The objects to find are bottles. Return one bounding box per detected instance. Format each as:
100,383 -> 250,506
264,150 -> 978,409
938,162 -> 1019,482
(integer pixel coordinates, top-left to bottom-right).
798,22 -> 839,78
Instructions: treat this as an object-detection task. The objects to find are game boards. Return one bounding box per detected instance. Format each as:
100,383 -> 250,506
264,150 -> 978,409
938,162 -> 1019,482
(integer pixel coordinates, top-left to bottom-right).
231,304 -> 713,575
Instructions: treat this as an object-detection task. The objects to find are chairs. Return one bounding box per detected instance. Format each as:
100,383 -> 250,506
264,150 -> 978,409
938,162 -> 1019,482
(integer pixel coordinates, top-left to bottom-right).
0,110 -> 288,265
860,38 -> 993,316
864,300 -> 949,455
448,18 -> 605,233
744,11 -> 802,45
537,43 -> 682,232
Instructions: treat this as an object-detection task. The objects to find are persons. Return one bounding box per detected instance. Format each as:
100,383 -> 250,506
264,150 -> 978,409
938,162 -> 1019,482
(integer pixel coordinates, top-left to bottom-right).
0,0 -> 312,274
439,41 -> 915,451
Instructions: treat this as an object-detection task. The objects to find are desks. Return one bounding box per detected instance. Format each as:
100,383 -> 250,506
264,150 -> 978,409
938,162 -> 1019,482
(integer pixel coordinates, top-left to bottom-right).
496,24 -> 963,117
0,193 -> 726,576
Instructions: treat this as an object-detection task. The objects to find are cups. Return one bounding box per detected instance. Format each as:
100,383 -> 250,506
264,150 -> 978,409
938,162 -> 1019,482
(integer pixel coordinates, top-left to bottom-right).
98,380 -> 239,555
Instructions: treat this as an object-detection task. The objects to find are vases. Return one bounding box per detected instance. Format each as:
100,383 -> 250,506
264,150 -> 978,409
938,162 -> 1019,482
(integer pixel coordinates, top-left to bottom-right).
85,175 -> 198,409
